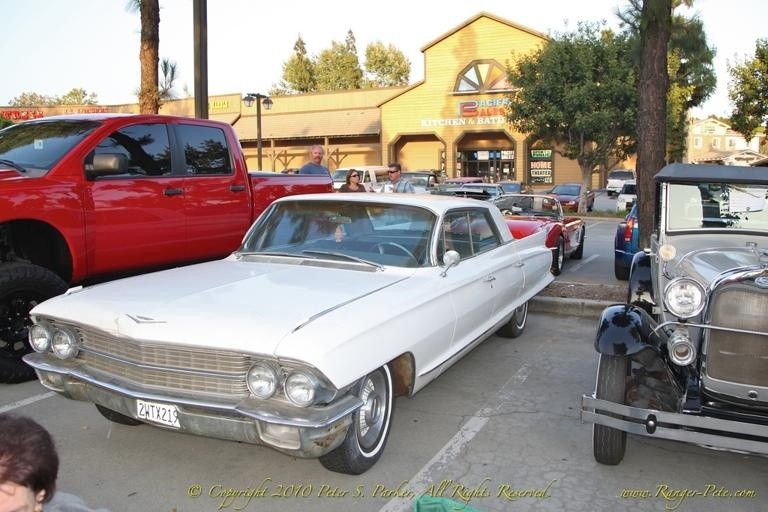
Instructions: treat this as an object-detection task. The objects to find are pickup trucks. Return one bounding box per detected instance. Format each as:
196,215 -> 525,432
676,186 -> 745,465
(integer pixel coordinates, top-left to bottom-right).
606,170 -> 634,196
0,115 -> 333,385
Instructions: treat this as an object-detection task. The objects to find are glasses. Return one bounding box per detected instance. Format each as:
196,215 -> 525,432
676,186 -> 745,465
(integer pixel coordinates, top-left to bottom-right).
388,170 -> 398,173
351,175 -> 359,177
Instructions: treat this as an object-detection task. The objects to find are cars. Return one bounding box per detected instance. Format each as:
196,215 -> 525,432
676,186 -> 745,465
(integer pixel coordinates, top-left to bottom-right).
401,170 -> 534,215
19,193 -> 557,476
541,183 -> 595,213
614,204 -> 640,281
443,194 -> 586,276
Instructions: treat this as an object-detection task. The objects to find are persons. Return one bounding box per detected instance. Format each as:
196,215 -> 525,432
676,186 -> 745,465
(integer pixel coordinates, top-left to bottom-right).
380,163 -> 414,225
299,145 -> 334,185
339,169 -> 366,222
0,412 -> 92,512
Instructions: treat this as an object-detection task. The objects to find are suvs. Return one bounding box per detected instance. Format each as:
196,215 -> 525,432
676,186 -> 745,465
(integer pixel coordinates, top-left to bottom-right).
616,180 -> 638,212
578,162 -> 768,465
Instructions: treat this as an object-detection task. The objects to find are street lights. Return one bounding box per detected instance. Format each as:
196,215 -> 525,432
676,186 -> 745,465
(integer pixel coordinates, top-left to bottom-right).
244,92 -> 272,172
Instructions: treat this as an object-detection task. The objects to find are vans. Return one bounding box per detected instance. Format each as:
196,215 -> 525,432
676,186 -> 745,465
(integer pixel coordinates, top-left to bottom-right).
331,165 -> 394,192
